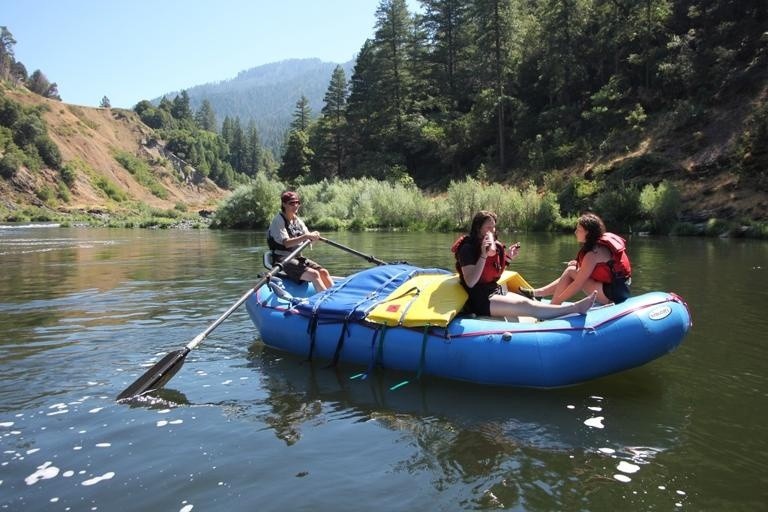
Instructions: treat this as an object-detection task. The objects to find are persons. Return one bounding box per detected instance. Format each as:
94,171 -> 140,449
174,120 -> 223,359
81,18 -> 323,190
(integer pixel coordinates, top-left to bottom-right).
265,188 -> 337,290
260,375 -> 323,448
450,208 -> 599,323
515,214 -> 633,322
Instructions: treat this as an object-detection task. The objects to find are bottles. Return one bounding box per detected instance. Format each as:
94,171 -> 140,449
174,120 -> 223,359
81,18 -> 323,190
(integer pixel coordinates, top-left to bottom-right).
485,230 -> 497,258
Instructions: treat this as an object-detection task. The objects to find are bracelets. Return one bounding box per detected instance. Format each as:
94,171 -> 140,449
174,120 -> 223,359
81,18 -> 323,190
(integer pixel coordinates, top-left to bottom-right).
479,254 -> 488,260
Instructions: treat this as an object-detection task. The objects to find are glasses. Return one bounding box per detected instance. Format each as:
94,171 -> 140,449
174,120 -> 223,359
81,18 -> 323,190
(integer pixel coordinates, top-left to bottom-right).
288,201 -> 300,205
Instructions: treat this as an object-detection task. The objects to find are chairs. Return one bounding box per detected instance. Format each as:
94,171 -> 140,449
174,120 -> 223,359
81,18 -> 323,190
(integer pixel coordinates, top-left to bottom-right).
263,251 -> 288,277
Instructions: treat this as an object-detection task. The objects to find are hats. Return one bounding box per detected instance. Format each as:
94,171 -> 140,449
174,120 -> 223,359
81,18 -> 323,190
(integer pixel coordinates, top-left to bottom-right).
282,191 -> 297,202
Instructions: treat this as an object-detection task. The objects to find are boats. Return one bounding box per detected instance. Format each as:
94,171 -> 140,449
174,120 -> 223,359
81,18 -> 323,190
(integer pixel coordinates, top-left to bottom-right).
248,335 -> 710,469
243,273 -> 695,392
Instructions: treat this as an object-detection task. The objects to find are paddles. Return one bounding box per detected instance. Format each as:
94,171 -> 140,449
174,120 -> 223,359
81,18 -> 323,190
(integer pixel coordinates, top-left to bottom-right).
118,241 -> 310,398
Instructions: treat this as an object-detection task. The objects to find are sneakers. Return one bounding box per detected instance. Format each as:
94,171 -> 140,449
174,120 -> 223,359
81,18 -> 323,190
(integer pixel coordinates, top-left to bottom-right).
518,286 -> 542,301
